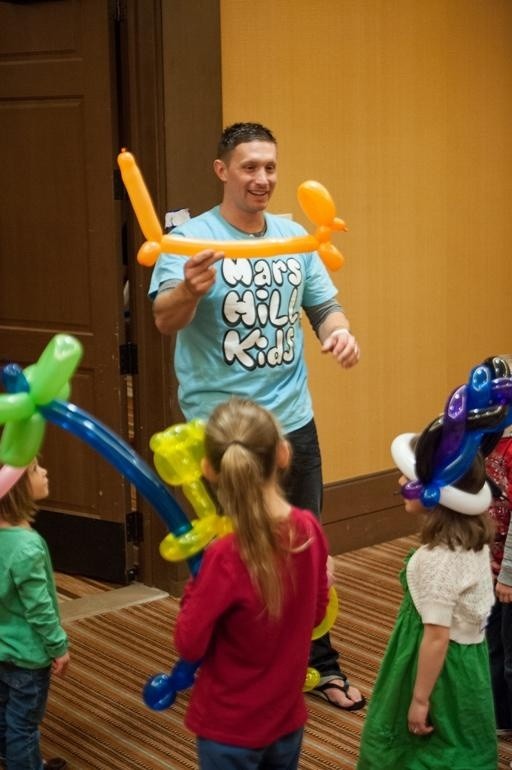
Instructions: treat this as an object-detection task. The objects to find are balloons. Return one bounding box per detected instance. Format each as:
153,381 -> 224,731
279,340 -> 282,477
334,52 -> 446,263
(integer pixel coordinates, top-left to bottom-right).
0,463 -> 32,500
0,332 -> 85,471
147,415 -> 339,694
113,145 -> 351,273
0,363 -> 205,712
386,349 -> 512,516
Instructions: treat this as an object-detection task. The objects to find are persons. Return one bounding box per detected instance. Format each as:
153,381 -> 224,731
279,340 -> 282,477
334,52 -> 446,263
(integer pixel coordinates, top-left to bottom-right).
143,116 -> 367,713
353,430 -> 501,768
0,447 -> 75,768
166,397 -> 338,768
480,423 -> 512,743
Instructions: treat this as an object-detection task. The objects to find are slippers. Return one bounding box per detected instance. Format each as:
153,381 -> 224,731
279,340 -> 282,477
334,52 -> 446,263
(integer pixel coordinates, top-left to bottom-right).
312,675 -> 366,710
